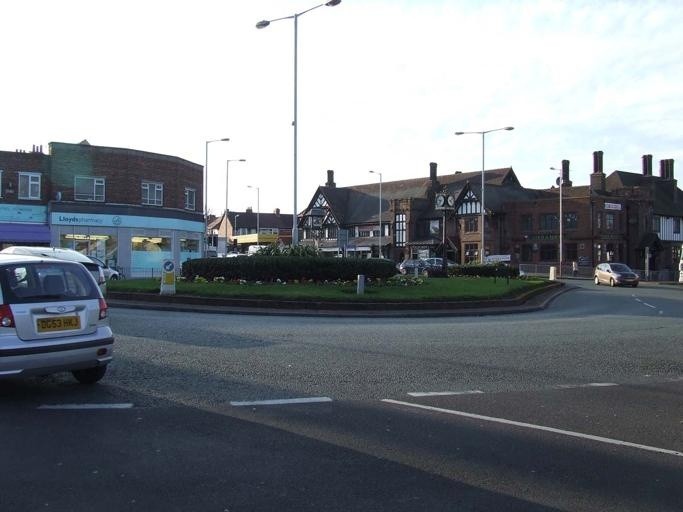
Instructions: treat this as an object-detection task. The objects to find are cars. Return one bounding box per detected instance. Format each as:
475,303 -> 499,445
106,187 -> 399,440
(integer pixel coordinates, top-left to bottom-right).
0,242 -> 124,394
399,254 -> 519,278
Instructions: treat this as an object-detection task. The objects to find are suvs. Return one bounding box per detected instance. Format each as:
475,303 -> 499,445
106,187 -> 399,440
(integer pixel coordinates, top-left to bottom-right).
593,261 -> 639,288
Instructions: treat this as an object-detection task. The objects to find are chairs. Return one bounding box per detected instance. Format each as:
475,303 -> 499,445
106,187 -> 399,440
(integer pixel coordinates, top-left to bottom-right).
44,274 -> 65,291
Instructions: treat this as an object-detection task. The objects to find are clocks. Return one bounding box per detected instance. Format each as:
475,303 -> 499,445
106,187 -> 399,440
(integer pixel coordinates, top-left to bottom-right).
447,195 -> 456,207
436,195 -> 445,207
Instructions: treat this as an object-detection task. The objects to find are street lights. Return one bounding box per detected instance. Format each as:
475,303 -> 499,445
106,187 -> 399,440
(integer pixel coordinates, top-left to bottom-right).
205,137 -> 231,255
548,166 -> 562,277
454,125 -> 514,265
234,214 -> 242,236
368,170 -> 382,259
254,0 -> 343,251
246,185 -> 259,247
225,158 -> 247,255
